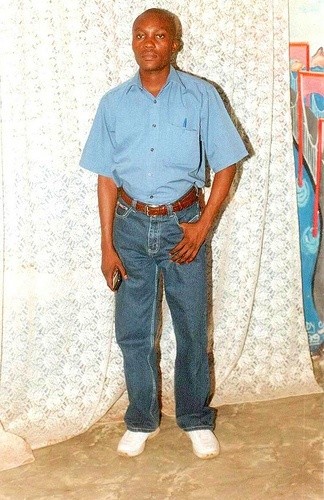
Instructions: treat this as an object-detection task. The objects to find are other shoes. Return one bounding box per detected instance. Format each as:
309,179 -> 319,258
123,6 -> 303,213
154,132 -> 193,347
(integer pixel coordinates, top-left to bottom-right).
116,427 -> 160,457
187,430 -> 220,459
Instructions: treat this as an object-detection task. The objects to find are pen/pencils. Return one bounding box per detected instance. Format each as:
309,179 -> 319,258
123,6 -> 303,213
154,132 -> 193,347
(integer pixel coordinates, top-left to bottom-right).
183,118 -> 186,128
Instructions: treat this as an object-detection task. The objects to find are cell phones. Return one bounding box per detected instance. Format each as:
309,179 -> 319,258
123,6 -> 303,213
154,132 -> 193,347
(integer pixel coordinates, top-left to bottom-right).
111,268 -> 122,290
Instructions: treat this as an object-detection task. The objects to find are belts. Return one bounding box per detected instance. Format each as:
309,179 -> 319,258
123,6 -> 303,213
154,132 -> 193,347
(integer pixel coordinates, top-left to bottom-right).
120,186 -> 196,216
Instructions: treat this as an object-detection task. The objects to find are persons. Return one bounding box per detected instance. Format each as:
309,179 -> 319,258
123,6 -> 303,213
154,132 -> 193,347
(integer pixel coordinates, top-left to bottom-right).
79,8 -> 247,460
